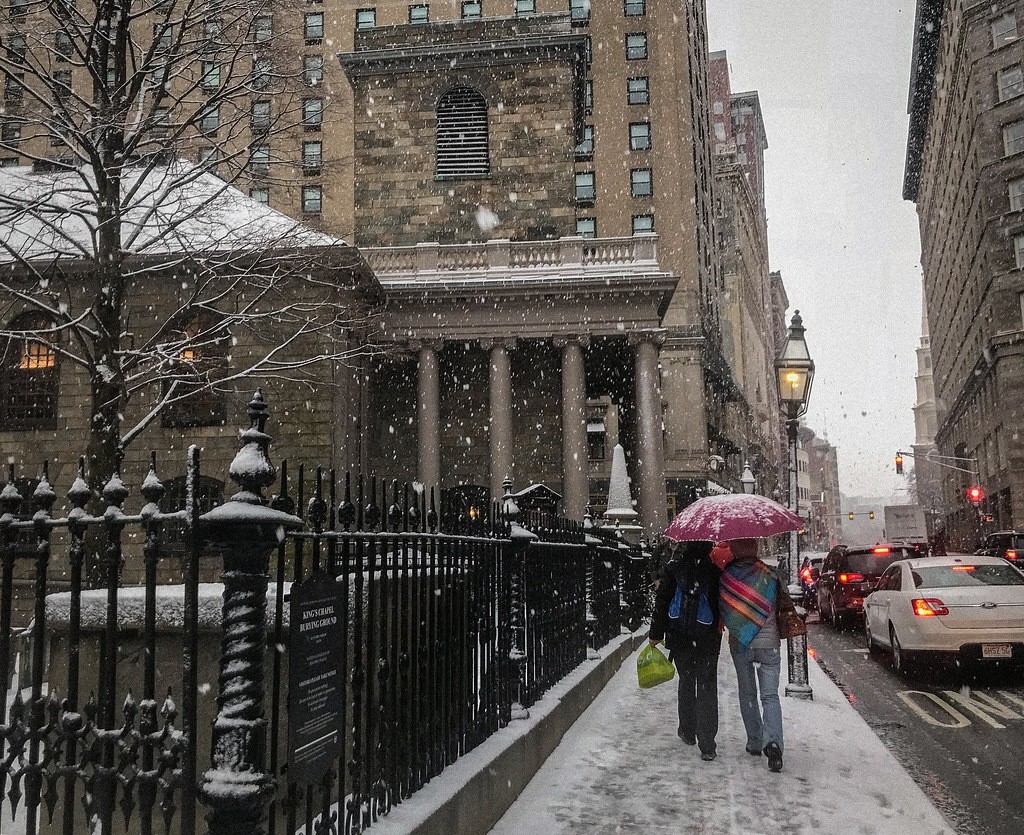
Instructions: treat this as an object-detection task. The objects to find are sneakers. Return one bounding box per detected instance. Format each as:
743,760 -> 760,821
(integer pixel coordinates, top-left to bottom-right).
746,745 -> 761,756
702,749 -> 717,760
762,741 -> 783,772
678,727 -> 696,745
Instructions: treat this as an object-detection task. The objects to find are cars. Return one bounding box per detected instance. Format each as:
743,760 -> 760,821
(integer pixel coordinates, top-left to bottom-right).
801,555 -> 828,606
862,554 -> 1024,675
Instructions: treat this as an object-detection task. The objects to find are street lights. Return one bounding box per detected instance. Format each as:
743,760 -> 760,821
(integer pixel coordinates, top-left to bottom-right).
772,310 -> 814,702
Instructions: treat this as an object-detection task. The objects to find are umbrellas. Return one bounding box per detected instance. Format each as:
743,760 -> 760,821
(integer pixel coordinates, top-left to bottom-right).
662,491 -> 806,542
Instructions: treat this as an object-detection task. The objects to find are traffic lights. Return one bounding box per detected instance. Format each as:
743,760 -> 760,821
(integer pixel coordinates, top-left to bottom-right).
895,457 -> 902,474
849,512 -> 853,520
869,511 -> 874,519
970,488 -> 980,511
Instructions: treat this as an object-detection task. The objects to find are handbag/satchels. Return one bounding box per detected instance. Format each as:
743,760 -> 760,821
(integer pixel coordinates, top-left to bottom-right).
718,558 -> 780,648
777,573 -> 805,639
636,639 -> 674,688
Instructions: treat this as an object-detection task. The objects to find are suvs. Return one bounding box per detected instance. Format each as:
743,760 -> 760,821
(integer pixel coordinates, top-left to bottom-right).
976,530 -> 1023,573
816,543 -> 904,628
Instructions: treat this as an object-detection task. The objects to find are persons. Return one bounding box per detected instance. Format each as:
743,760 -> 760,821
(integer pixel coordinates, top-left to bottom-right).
721,539 -> 790,772
648,541 -> 723,761
